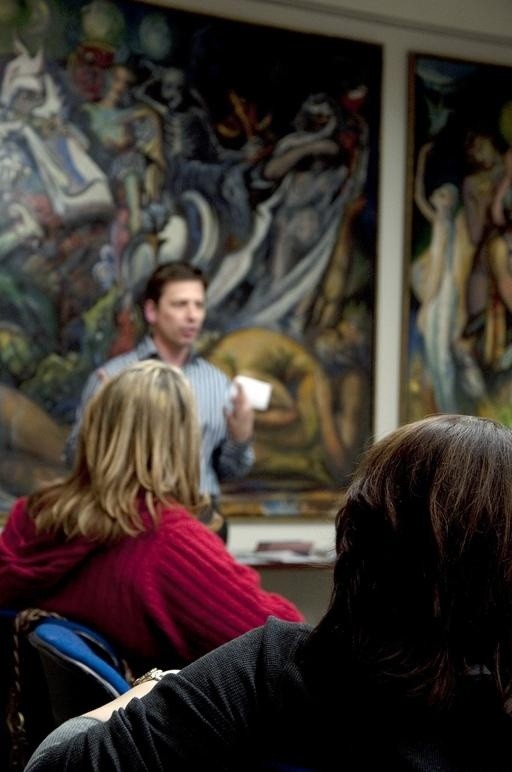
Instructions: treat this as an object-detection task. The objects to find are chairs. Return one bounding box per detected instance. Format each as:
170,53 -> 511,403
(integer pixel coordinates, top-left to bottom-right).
27,624 -> 132,725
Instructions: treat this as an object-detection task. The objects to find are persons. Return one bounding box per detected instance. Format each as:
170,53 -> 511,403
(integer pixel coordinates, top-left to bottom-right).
66,261 -> 255,551
27,414 -> 511,771
0,360 -> 303,669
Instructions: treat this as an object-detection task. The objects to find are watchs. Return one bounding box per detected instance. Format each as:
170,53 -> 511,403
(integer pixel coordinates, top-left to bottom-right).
133,667 -> 165,686
